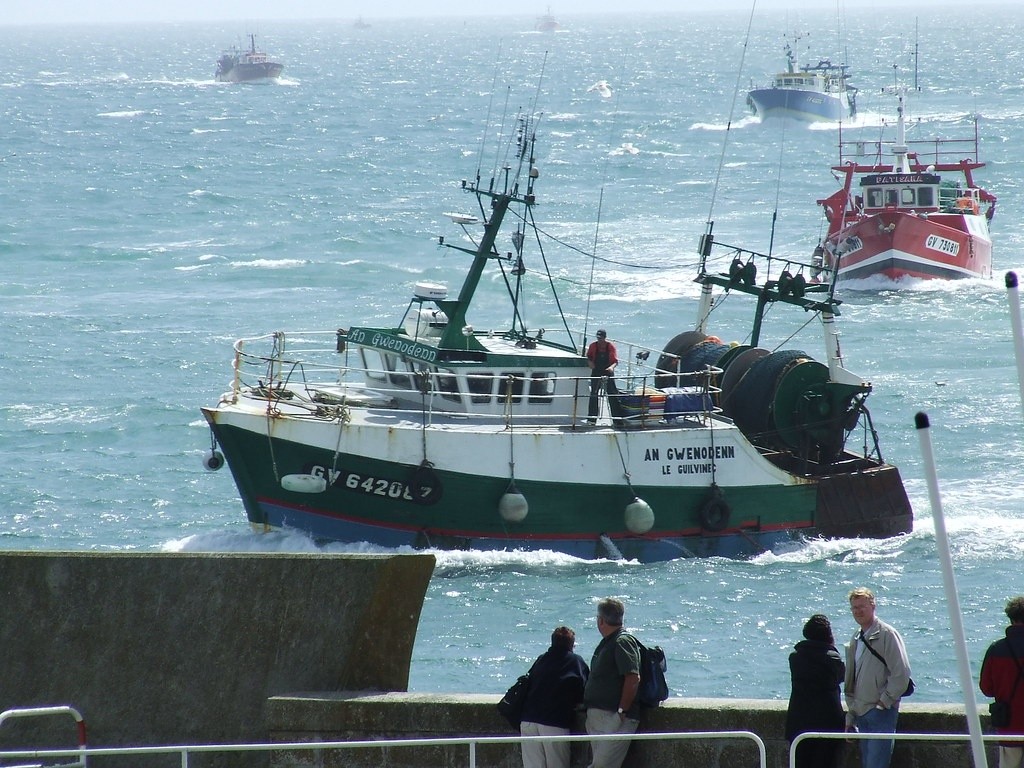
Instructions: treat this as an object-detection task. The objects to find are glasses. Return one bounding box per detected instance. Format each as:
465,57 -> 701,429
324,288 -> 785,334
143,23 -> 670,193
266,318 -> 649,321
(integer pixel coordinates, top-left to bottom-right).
850,605 -> 869,612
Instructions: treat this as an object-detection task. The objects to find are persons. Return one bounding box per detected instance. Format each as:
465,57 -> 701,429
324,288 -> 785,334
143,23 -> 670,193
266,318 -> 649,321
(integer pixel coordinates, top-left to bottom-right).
979,597 -> 1024,768
586,329 -> 618,426
582,597 -> 644,768
843,587 -> 911,767
787,615 -> 847,768
521,626 -> 589,767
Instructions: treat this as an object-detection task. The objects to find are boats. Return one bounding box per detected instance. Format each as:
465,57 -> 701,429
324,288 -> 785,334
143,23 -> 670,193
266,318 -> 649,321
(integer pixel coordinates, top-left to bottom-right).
197,4 -> 920,567
216,33 -> 284,83
815,61 -> 996,292
745,35 -> 858,121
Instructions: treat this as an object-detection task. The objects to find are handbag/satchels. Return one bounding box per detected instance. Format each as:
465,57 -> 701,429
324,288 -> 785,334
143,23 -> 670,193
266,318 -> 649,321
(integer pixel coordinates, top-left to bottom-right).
989,699 -> 1011,727
902,679 -> 916,696
498,652 -> 548,729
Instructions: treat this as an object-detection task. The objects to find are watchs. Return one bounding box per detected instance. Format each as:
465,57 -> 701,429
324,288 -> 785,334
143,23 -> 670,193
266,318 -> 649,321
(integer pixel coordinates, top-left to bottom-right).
618,708 -> 626,713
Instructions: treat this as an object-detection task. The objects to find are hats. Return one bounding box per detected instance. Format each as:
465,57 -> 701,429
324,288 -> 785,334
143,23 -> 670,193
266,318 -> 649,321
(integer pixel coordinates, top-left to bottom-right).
596,330 -> 606,337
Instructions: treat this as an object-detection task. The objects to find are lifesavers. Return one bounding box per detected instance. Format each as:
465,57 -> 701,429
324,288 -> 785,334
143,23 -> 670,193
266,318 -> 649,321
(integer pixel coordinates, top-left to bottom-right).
698,497 -> 730,532
280,474 -> 327,494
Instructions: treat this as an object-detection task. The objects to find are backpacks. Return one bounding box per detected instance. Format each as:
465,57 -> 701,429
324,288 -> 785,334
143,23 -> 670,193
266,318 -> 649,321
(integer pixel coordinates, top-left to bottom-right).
612,633 -> 668,715
